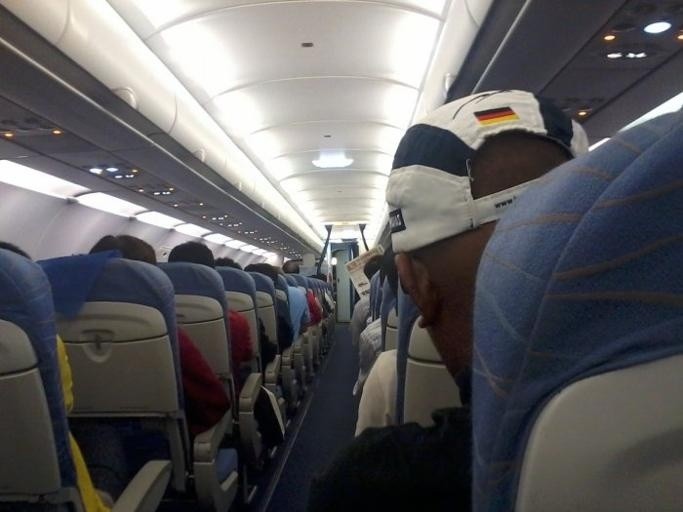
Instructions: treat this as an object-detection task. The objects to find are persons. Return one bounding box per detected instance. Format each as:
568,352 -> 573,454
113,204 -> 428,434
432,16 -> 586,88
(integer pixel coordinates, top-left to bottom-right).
307,89 -> 589,512
352,244 -> 399,397
347,254 -> 384,347
1,232 -> 336,512
354,269 -> 399,438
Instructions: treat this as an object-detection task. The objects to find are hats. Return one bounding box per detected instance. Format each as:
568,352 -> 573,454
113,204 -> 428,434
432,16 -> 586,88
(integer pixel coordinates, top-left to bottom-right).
383,88 -> 591,254
